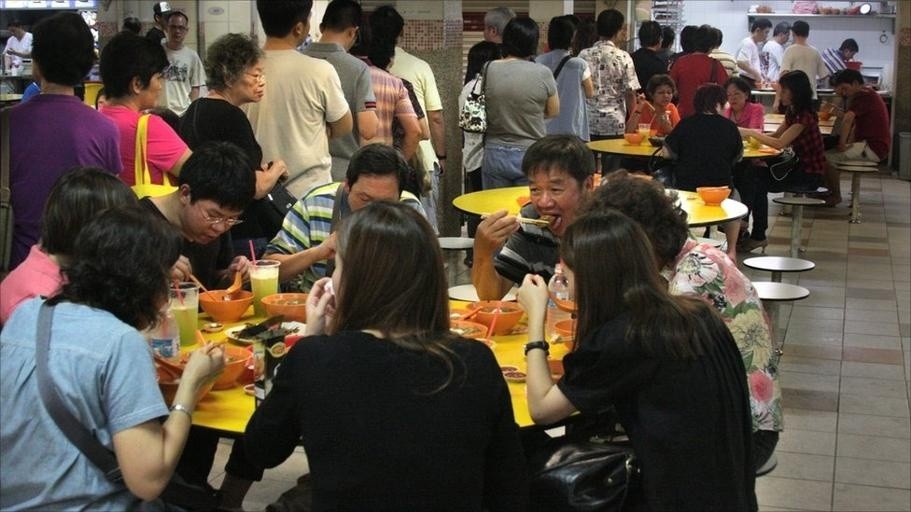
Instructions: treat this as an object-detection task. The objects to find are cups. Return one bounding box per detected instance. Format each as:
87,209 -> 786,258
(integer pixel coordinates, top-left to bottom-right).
639,123 -> 651,143
248,259 -> 281,317
168,282 -> 202,352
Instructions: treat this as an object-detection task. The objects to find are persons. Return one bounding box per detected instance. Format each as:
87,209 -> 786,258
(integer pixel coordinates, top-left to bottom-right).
456,8 -> 892,303
580,170 -> 783,473
1,202 -> 229,510
221,196 -> 520,512
1,0 -> 446,330
521,209 -> 761,511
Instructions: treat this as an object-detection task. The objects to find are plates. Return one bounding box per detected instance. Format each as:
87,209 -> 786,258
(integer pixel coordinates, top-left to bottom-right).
243,384 -> 256,395
500,366 -> 519,376
225,320 -> 307,345
505,371 -> 527,383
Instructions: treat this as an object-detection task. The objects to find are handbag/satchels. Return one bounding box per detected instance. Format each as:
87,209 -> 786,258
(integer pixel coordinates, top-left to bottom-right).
459,59 -> 492,133
256,181 -> 298,241
130,113 -> 180,201
768,148 -> 799,181
523,439 -> 638,512
648,147 -> 674,187
153,473 -> 223,511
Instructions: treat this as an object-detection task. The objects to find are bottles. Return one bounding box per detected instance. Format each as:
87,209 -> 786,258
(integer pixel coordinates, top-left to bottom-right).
148,309 -> 181,384
546,264 -> 571,344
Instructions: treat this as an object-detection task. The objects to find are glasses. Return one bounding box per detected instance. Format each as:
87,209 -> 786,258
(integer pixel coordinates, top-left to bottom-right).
353,21 -> 362,47
243,72 -> 264,81
196,200 -> 247,226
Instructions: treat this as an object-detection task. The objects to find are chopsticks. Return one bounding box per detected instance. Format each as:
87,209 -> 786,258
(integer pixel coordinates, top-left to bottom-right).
195,328 -> 215,356
190,274 -> 218,301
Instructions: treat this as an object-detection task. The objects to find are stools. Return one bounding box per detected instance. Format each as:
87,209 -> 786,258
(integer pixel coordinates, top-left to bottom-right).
436,234 -> 474,281
737,159 -> 881,356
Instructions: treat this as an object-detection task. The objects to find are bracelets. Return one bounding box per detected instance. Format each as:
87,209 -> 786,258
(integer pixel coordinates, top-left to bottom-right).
169,404 -> 193,422
524,341 -> 550,356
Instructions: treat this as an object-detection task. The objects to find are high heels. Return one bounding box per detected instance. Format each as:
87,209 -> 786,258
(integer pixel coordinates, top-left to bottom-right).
719,220 -> 749,232
736,237 -> 768,253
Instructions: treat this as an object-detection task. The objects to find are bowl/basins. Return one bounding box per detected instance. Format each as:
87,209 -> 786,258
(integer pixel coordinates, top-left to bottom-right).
466,300 -> 524,336
261,293 -> 309,322
199,289 -> 255,323
625,133 -> 646,146
209,346 -> 255,390
449,308 -> 478,321
548,359 -> 565,384
754,80 -> 777,91
696,186 -> 733,206
449,320 -> 489,339
554,319 -> 578,351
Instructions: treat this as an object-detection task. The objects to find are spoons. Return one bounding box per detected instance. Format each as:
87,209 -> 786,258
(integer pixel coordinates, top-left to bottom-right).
547,290 -> 579,312
153,357 -> 183,380
226,271 -> 243,294
